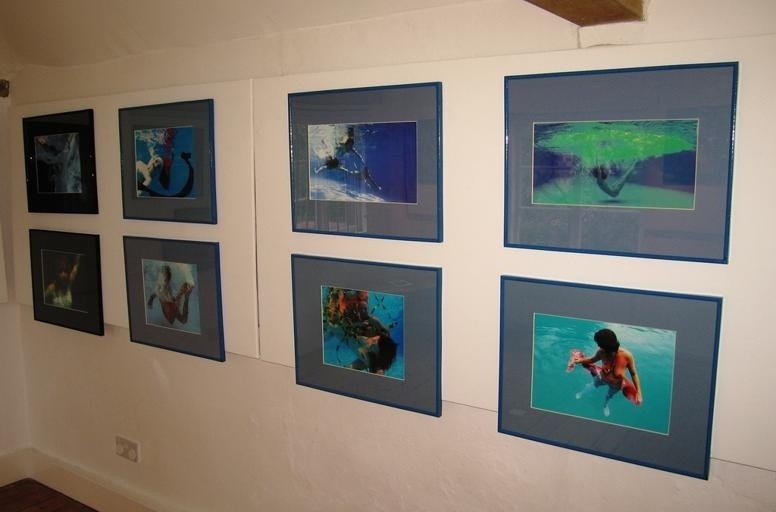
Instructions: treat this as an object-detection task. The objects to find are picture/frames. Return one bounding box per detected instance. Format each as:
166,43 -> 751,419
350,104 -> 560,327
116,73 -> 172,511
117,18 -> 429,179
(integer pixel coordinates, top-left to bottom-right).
503,62 -> 739,263
22,108 -> 99,214
29,229 -> 104,336
118,98 -> 217,223
286,82 -> 443,243
122,235 -> 225,362
497,274 -> 723,481
291,253 -> 442,418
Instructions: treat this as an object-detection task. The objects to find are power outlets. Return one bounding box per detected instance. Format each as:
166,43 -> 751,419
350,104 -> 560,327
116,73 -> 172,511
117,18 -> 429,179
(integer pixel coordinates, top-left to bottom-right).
114,434 -> 142,465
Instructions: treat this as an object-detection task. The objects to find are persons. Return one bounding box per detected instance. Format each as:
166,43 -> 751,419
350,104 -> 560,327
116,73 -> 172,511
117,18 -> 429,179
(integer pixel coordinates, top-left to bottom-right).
148,265 -> 194,326
314,157 -> 360,176
592,153 -> 640,197
574,329 -> 642,417
44,255 -> 81,309
136,157 -> 164,197
336,127 -> 364,162
349,317 -> 398,375
350,165 -> 382,191
